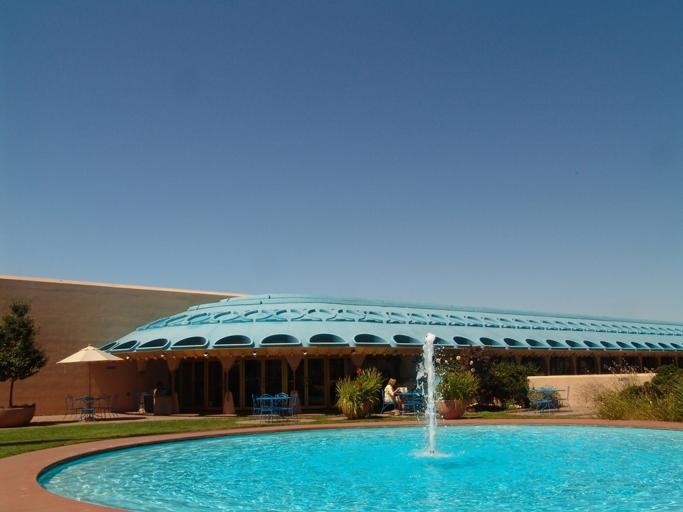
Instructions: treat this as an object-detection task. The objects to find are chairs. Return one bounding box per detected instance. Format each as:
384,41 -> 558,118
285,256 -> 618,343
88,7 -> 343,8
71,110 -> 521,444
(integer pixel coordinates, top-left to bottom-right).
380,388 -> 422,423
251,392 -> 298,423
64,393 -> 119,422
524,385 -> 564,416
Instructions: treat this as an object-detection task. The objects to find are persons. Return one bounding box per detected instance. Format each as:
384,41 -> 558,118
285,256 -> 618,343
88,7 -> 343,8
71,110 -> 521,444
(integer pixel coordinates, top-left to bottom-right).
384,377 -> 401,416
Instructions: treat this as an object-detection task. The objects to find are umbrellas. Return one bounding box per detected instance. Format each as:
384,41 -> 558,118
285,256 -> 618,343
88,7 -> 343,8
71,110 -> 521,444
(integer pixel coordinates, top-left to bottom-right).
54,343 -> 126,417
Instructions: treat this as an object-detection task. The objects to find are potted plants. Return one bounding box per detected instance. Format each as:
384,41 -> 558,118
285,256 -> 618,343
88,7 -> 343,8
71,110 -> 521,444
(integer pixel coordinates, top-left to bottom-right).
333,375 -> 373,419
433,366 -> 478,419
